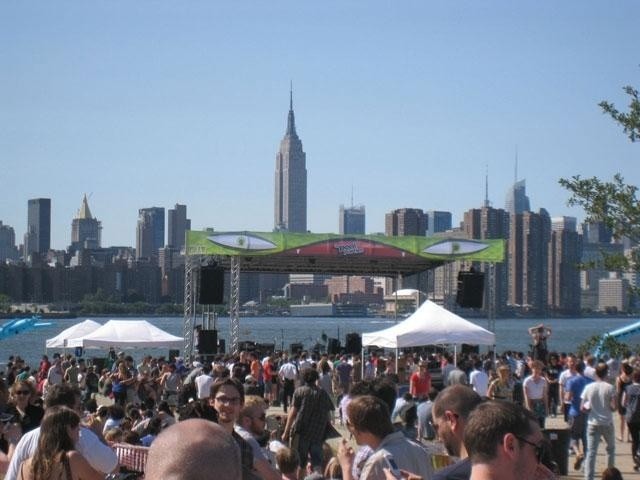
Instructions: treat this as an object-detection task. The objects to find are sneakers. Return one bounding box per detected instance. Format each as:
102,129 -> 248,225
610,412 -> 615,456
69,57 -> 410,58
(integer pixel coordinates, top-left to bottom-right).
574,453 -> 585,471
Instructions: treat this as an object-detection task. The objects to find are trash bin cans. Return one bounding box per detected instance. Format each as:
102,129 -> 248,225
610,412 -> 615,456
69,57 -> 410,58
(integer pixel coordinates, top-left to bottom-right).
540,429 -> 572,476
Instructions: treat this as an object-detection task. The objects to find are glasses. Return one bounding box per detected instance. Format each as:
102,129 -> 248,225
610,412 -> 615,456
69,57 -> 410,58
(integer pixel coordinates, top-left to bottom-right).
15,390 -> 31,395
249,414 -> 266,421
514,436 -> 547,463
214,395 -> 242,406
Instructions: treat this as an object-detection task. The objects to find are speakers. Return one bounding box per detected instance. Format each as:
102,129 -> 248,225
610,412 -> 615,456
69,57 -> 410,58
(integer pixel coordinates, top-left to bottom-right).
199,330 -> 217,354
458,271 -> 484,309
199,265 -> 223,305
328,338 -> 339,354
346,334 -> 358,354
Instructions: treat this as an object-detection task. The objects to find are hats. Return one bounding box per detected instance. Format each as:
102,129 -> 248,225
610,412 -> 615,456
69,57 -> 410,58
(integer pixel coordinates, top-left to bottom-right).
244,374 -> 259,383
